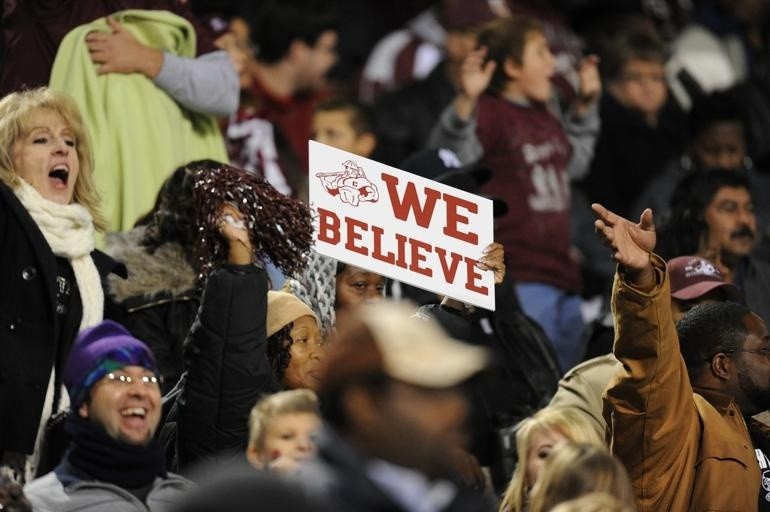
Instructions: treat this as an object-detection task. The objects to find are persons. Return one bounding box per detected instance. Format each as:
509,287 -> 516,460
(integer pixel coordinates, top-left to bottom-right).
0,1 -> 769,512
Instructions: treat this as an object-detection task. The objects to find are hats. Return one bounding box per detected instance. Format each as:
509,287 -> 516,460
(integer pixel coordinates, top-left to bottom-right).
60,321 -> 156,414
266,290 -> 318,339
667,256 -> 744,305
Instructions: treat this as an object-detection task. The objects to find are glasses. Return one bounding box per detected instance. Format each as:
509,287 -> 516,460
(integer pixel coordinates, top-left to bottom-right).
105,372 -> 163,389
